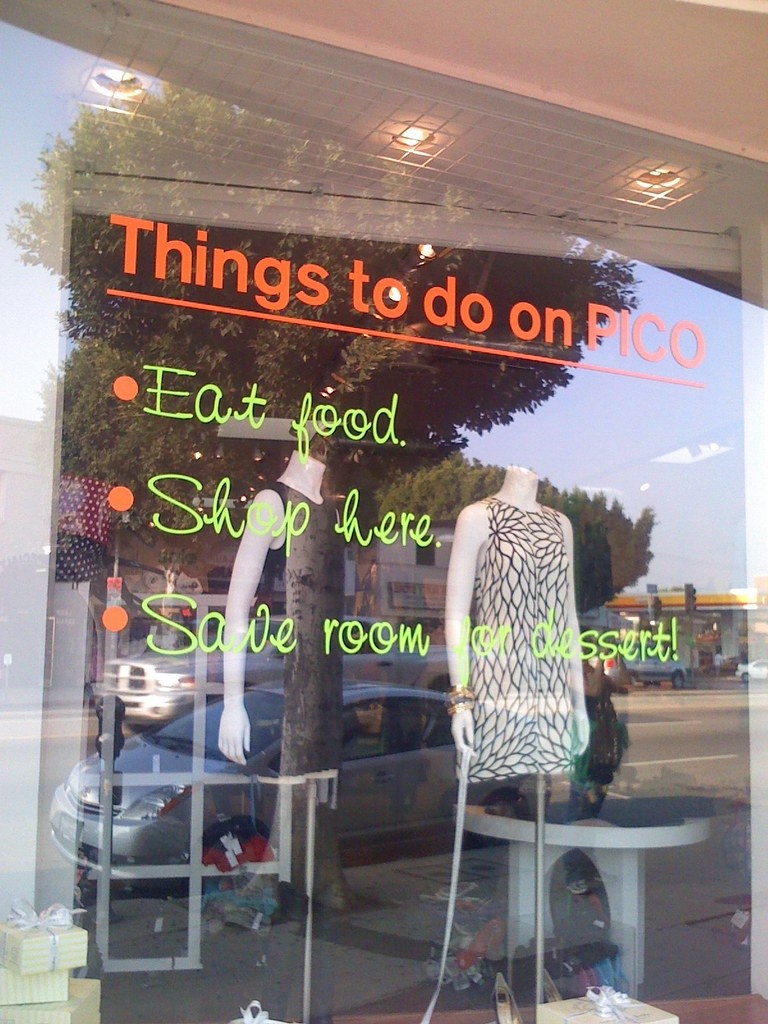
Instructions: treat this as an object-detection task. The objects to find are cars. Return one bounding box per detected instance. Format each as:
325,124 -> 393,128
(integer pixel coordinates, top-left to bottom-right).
587,640 -> 687,691
735,658 -> 768,684
48,680 -> 551,892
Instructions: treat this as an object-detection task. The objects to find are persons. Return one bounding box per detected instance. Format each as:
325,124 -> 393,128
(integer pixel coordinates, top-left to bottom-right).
421,465 -> 590,1024
219,451 -> 345,1024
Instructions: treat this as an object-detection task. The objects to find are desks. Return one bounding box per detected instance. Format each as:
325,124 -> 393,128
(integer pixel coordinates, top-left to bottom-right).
453,802 -> 711,1000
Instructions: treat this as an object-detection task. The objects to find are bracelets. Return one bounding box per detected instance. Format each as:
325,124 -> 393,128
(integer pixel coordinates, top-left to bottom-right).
445,685 -> 474,715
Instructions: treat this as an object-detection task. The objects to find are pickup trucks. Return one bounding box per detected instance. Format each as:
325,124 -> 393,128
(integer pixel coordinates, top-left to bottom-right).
94,615 -> 451,732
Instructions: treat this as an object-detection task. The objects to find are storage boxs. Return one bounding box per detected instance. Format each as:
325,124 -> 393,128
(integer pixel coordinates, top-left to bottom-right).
0,898 -> 102,1024
536,985 -> 681,1023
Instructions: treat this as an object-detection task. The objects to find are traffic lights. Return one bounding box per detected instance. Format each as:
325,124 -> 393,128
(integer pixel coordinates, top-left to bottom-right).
653,597 -> 662,621
684,585 -> 697,614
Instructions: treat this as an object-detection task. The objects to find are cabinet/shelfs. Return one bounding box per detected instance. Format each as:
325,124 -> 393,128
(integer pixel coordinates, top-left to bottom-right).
84,589 -> 307,973
